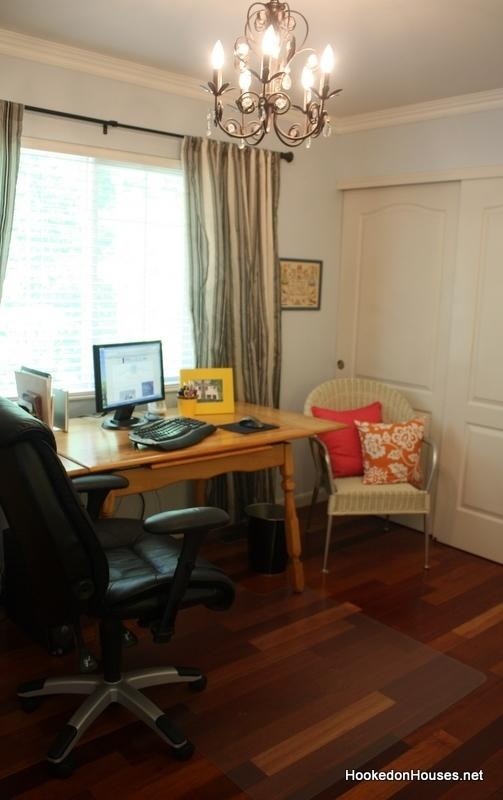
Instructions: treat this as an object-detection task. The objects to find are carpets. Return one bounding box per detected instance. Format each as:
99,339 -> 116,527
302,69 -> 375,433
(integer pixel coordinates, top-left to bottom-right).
171,612 -> 489,799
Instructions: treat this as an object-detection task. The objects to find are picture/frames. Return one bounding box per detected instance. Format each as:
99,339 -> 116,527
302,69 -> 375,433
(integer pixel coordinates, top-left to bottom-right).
179,368 -> 236,415
278,257 -> 323,311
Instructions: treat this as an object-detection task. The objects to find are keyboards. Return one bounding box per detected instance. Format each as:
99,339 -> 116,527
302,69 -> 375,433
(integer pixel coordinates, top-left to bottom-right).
127,417 -> 215,449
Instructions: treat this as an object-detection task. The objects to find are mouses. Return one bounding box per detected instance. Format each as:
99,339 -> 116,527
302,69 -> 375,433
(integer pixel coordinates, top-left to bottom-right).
240,415 -> 263,430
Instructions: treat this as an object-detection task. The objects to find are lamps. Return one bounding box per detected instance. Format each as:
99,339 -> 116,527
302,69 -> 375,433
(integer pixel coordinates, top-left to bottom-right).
197,0 -> 343,152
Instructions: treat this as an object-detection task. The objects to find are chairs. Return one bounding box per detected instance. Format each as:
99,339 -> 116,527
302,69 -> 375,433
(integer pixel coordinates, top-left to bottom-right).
2,399 -> 238,772
301,376 -> 444,575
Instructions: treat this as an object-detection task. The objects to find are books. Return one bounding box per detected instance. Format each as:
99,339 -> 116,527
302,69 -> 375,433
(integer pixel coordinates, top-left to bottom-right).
15,366 -> 68,433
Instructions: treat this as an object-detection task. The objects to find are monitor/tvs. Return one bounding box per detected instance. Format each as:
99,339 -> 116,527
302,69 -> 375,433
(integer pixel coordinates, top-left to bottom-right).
91,339 -> 166,429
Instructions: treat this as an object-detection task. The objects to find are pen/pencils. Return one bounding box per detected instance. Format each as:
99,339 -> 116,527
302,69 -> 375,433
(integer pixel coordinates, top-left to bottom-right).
178,385 -> 198,398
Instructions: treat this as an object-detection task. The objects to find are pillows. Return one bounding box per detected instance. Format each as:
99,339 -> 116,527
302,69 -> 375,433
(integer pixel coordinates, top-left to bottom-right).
310,400 -> 384,479
353,418 -> 427,486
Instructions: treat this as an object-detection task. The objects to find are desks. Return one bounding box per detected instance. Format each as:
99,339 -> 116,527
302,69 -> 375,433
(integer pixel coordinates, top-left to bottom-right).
33,391 -> 348,596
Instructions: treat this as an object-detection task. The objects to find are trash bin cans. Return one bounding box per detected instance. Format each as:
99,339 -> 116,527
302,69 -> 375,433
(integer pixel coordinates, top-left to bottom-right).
244,503 -> 288,575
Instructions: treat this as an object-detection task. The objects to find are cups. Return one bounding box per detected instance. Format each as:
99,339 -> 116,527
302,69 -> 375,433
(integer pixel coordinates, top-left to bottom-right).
146,398 -> 167,416
